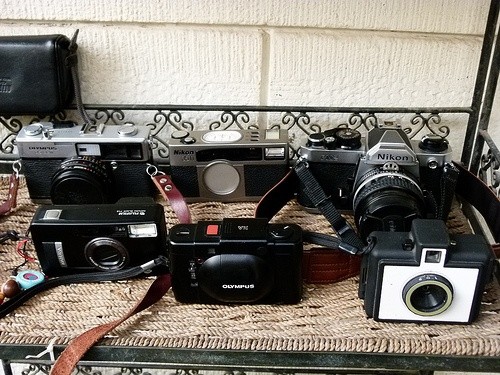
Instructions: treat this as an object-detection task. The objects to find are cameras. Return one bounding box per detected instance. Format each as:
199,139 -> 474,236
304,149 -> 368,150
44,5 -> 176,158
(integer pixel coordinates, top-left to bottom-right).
15,119 -> 489,328
